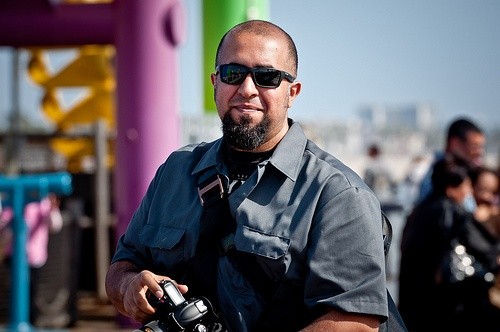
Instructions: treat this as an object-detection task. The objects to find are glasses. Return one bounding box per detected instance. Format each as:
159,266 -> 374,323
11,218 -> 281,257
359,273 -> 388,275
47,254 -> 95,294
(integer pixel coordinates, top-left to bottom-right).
216,63 -> 294,87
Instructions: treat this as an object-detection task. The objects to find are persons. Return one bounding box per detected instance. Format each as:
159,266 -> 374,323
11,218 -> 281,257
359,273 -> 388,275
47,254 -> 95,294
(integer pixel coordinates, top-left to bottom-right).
0,153 -> 100,331
399,117 -> 500,331
104,20 -> 408,331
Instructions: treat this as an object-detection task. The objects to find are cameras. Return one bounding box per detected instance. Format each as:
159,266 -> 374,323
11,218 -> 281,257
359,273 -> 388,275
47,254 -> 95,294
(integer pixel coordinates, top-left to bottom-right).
143,279 -> 218,332
